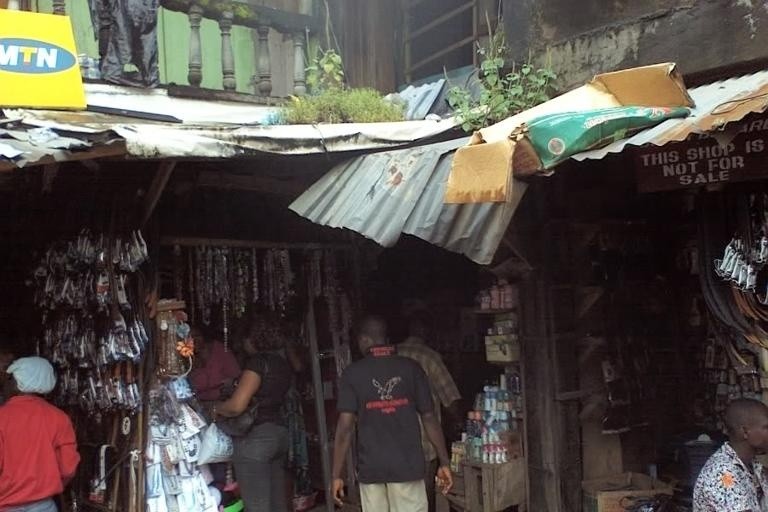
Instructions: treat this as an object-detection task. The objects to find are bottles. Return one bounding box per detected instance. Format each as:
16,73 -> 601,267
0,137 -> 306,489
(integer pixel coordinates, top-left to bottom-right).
448,275 -> 523,473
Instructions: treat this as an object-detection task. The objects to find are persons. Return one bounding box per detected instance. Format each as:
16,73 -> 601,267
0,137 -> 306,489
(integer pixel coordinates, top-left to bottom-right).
181,293 -> 467,512
693,398 -> 768,512
0,354 -> 81,512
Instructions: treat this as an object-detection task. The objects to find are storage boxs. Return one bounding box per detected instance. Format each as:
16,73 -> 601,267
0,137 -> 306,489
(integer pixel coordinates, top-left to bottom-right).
581,473 -> 671,511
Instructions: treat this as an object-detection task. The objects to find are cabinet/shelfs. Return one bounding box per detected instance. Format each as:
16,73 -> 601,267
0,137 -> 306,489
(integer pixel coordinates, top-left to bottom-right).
435,308 -> 528,512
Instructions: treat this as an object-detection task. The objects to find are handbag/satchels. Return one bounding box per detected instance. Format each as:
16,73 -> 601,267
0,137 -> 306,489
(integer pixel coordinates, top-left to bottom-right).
216,407 -> 255,436
